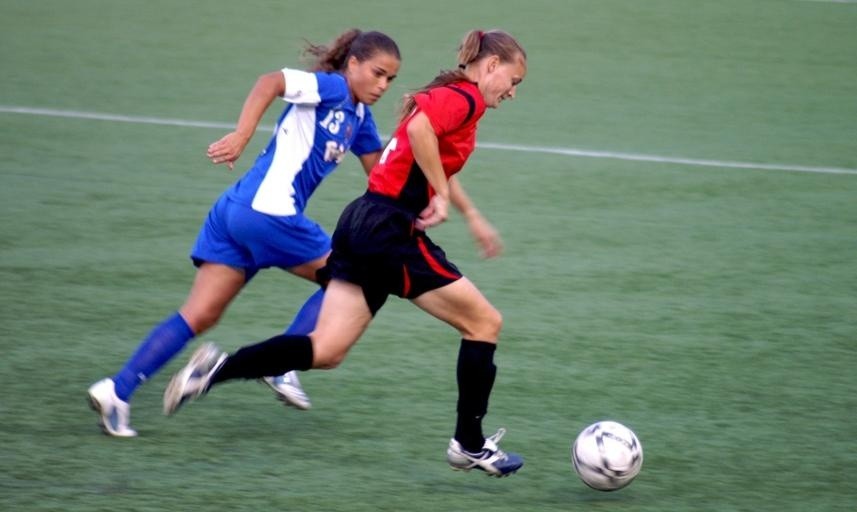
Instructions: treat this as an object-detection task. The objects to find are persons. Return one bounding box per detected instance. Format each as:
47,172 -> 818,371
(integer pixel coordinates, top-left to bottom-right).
87,31 -> 401,437
162,30 -> 526,479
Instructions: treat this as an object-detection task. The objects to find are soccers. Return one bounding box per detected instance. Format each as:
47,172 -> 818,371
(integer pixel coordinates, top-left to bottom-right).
571,421 -> 643,491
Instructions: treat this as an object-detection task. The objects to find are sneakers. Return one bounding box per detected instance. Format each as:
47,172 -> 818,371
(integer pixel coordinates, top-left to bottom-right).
259,368 -> 314,412
445,427 -> 525,478
87,375 -> 140,438
161,341 -> 228,418
